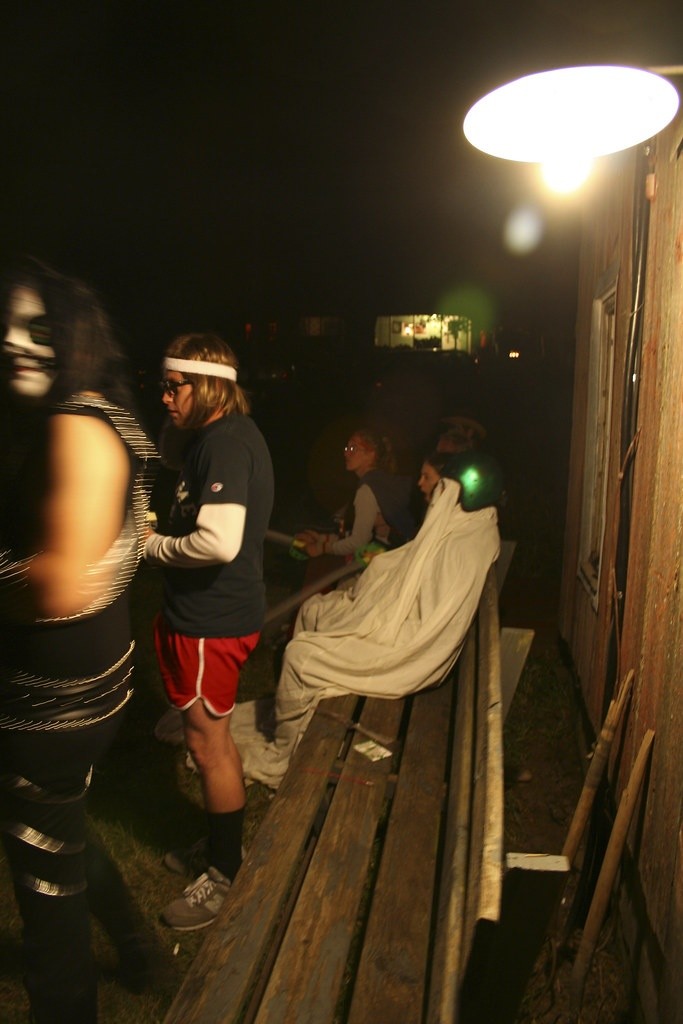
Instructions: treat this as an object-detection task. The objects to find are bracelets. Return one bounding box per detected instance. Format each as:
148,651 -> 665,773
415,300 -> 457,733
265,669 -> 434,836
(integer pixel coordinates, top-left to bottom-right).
323,543 -> 327,555
326,533 -> 330,542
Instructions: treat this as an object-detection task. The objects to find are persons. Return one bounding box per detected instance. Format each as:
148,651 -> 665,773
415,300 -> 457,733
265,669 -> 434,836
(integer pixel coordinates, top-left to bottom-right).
0,245 -> 182,1024
139,332 -> 500,931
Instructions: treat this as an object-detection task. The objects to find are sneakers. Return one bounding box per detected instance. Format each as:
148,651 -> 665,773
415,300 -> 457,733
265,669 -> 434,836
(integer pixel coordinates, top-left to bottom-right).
160,866 -> 237,930
165,840 -> 249,874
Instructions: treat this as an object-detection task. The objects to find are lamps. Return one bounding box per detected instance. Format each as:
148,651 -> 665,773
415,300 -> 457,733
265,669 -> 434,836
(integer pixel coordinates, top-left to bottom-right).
464,61 -> 683,165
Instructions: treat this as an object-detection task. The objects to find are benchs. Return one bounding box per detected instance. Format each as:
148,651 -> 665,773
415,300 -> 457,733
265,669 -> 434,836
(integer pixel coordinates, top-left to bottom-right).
161,539 -> 570,1024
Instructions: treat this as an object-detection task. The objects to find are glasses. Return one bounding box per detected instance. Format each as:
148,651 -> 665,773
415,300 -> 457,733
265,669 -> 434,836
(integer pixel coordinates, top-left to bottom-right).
155,377 -> 195,397
343,442 -> 366,456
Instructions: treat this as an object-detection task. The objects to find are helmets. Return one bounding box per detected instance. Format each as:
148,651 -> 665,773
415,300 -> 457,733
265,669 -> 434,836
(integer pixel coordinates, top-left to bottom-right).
447,444 -> 516,511
